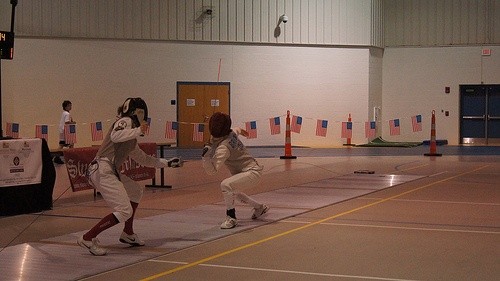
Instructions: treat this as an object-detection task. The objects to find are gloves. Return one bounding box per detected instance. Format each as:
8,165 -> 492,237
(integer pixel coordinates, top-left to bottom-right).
202,146 -> 212,157
167,157 -> 180,168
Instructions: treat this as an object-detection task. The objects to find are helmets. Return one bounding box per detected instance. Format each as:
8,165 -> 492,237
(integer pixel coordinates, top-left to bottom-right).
121,97 -> 148,136
208,113 -> 231,145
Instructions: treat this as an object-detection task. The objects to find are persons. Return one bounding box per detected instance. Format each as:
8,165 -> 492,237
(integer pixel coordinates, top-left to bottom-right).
202,112 -> 270,228
54,100 -> 76,164
77,98 -> 180,255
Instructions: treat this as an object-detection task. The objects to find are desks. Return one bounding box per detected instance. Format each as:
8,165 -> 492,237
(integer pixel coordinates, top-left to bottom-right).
48,142 -> 176,199
0,138 -> 56,217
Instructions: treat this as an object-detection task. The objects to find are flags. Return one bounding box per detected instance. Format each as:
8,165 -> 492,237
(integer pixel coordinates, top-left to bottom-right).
64,124 -> 77,144
390,119 -> 400,136
245,121 -> 257,139
412,115 -> 422,132
6,123 -> 19,138
316,119 -> 327,137
36,124 -> 48,141
341,122 -> 352,138
90,122 -> 103,142
165,120 -> 178,140
270,117 -> 280,135
145,118 -> 151,136
365,122 -> 376,138
291,115 -> 302,133
192,123 -> 204,142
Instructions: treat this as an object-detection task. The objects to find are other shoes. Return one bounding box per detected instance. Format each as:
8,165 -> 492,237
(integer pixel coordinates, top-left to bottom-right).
53,158 -> 64,164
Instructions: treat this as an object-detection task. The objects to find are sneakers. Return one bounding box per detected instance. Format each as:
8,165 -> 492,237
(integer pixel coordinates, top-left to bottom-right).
77,235 -> 106,255
251,205 -> 268,219
119,231 -> 145,246
221,216 -> 238,228
